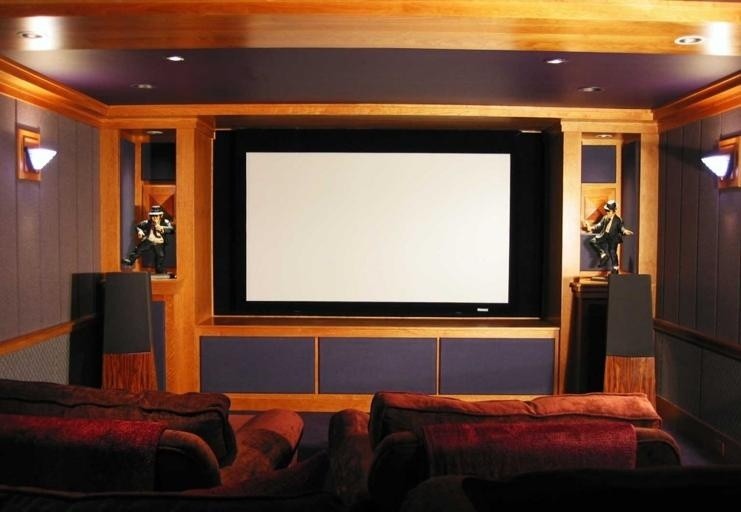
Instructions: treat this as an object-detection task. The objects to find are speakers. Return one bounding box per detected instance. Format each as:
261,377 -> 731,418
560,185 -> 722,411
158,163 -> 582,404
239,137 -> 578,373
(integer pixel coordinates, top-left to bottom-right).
101,269 -> 153,354
604,273 -> 655,358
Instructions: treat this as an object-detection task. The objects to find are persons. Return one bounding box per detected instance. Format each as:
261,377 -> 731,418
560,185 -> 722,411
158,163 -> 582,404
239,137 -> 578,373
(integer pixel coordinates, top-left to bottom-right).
122,205 -> 174,274
582,200 -> 634,278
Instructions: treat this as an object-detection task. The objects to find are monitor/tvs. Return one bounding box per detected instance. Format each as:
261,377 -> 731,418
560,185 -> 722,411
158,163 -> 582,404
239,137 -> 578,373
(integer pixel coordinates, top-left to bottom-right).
212,129 -> 548,317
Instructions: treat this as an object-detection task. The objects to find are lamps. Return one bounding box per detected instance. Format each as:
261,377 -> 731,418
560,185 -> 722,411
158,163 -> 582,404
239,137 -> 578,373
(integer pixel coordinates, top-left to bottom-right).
701,136 -> 741,189
17,128 -> 57,181
